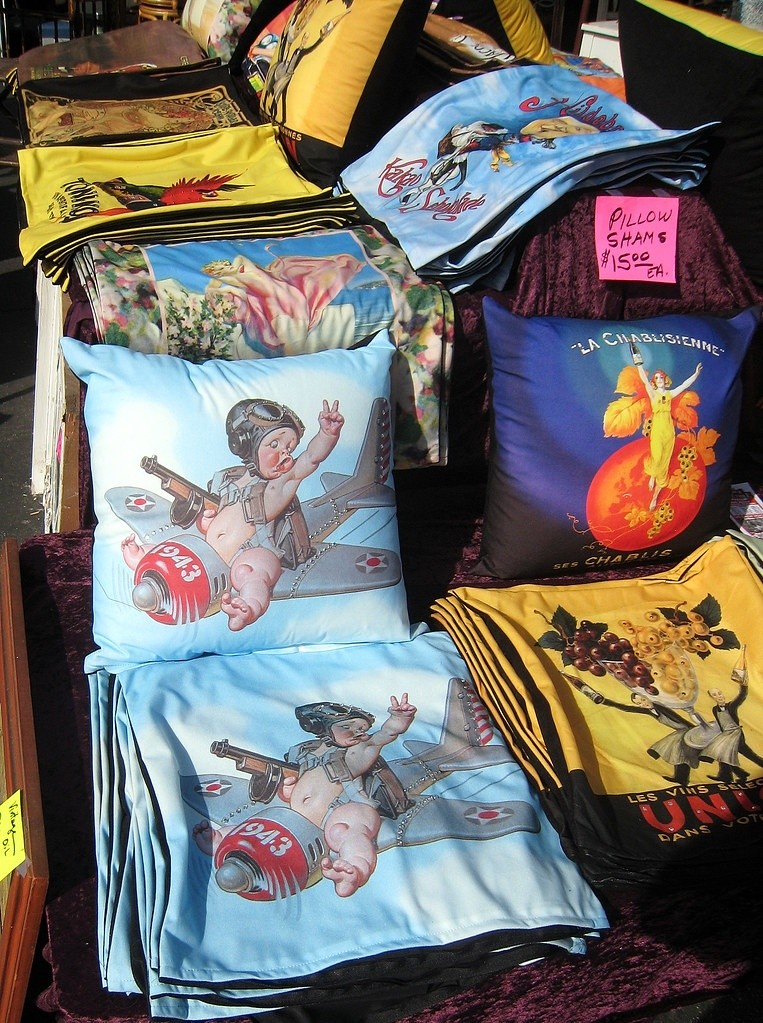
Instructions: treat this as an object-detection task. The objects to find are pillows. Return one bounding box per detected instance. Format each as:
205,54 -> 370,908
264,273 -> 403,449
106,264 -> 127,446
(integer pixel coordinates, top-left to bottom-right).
55,326 -> 435,674
180,0 -> 280,75
460,296 -> 757,579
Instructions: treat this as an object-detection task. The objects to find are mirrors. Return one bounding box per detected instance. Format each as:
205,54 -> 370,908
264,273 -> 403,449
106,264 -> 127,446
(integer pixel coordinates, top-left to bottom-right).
260,1 -> 434,194
